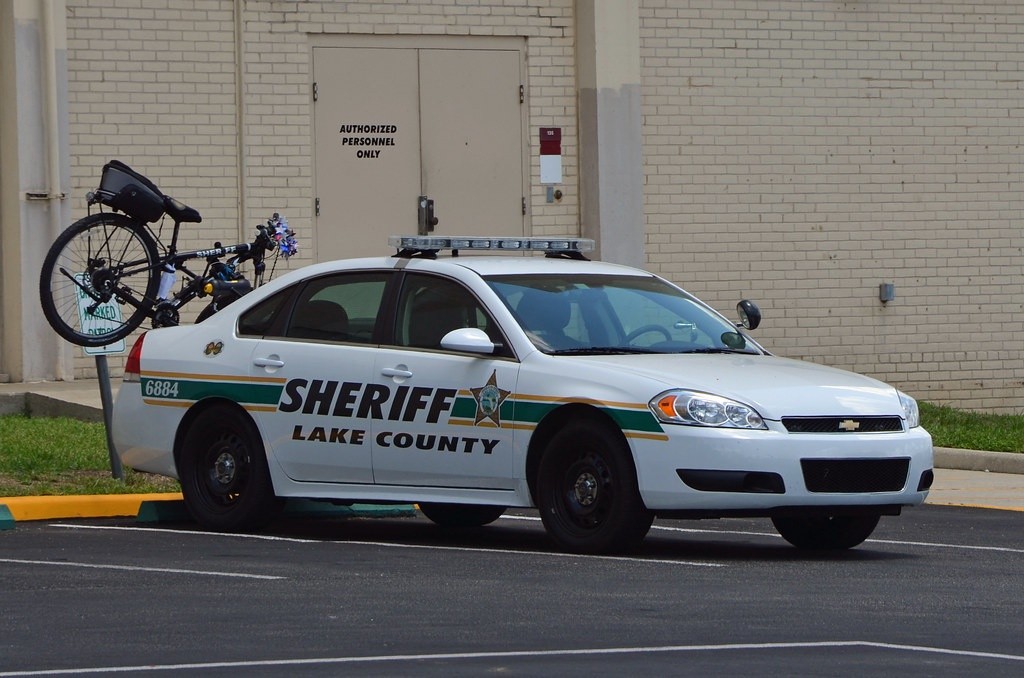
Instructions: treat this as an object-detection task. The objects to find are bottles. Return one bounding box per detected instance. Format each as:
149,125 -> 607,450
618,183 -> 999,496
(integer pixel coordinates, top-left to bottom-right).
158,253 -> 177,302
270,212 -> 292,254
203,279 -> 250,294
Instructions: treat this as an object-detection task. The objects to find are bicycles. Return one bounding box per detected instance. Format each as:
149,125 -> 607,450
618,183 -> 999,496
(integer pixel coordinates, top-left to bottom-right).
39,160 -> 300,346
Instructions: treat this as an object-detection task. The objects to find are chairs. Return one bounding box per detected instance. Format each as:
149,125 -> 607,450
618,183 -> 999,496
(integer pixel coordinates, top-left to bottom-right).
516,286 -> 588,349
304,300 -> 352,339
409,300 -> 466,349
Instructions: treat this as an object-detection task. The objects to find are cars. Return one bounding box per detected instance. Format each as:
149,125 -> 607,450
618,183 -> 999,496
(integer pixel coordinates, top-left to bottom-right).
107,234 -> 934,559
